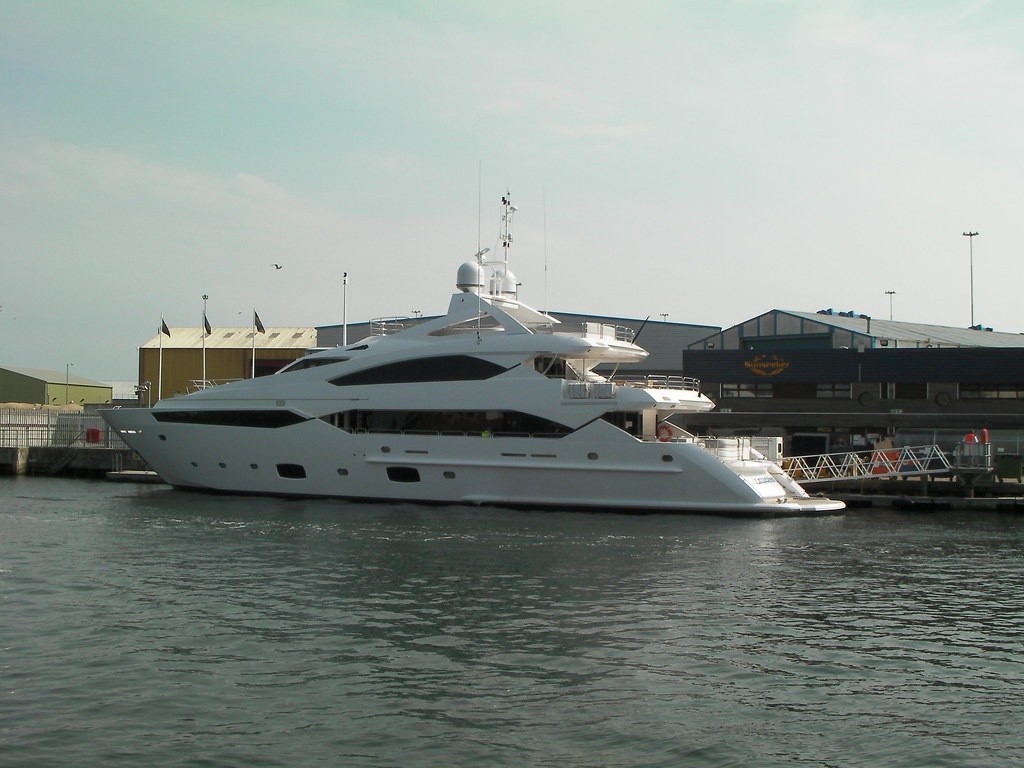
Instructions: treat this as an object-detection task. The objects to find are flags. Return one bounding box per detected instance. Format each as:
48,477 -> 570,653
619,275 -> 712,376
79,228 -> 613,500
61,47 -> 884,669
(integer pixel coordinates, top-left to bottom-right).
255,311 -> 265,334
162,318 -> 171,338
205,314 -> 212,335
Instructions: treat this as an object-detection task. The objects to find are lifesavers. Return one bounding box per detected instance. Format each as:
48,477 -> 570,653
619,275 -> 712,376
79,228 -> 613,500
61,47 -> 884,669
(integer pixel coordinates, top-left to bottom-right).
656,424 -> 673,442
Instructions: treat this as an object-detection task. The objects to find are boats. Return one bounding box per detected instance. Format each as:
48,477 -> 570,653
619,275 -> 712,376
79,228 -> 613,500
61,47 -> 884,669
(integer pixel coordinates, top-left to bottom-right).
96,164 -> 847,519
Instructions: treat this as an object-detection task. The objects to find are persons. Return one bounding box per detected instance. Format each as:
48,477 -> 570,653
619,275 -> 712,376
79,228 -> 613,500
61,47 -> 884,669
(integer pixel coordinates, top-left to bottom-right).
961,429 -> 979,468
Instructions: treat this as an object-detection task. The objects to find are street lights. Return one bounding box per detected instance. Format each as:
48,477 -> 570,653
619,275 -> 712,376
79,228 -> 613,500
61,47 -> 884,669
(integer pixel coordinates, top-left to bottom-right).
66,363 -> 74,404
962,232 -> 979,326
885,291 -> 896,321
